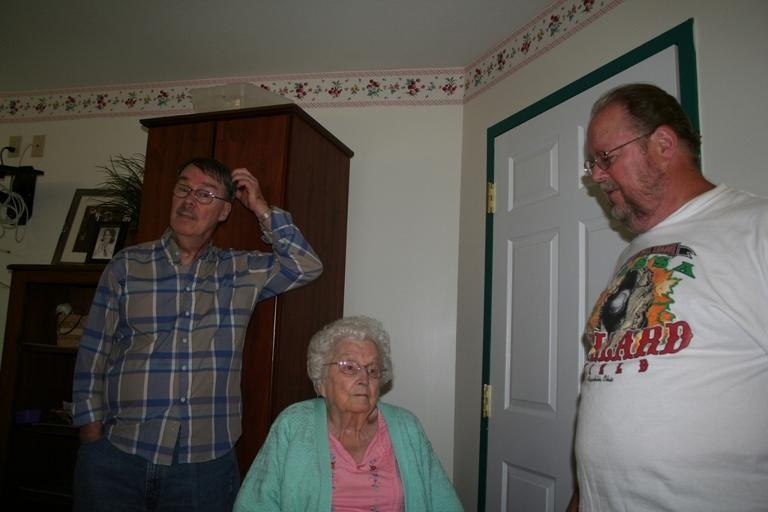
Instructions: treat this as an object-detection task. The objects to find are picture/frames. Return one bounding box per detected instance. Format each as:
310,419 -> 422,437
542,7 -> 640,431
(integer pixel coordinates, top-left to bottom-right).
50,188 -> 135,266
83,221 -> 130,263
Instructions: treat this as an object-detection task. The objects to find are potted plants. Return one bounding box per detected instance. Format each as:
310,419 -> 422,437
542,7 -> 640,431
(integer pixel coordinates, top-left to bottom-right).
88,155 -> 146,246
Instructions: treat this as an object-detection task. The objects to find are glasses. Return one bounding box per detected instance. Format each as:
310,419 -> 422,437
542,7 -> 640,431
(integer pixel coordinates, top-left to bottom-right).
582,131 -> 651,178
323,359 -> 388,379
173,182 -> 229,206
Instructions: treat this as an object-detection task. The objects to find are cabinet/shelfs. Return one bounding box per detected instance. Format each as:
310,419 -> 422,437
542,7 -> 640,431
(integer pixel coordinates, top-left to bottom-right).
134,102 -> 355,473
1,261 -> 108,511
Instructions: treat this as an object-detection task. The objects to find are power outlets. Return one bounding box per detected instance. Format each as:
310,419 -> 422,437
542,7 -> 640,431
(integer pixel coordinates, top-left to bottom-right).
6,134 -> 22,159
31,135 -> 45,157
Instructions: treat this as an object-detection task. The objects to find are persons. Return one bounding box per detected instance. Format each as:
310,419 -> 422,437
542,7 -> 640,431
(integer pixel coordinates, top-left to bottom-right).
65,157 -> 325,512
95,228 -> 116,256
566,82 -> 768,511
230,316 -> 465,512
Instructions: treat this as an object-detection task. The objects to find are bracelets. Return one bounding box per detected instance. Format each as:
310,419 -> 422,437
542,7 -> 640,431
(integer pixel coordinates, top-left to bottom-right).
257,208 -> 275,225
